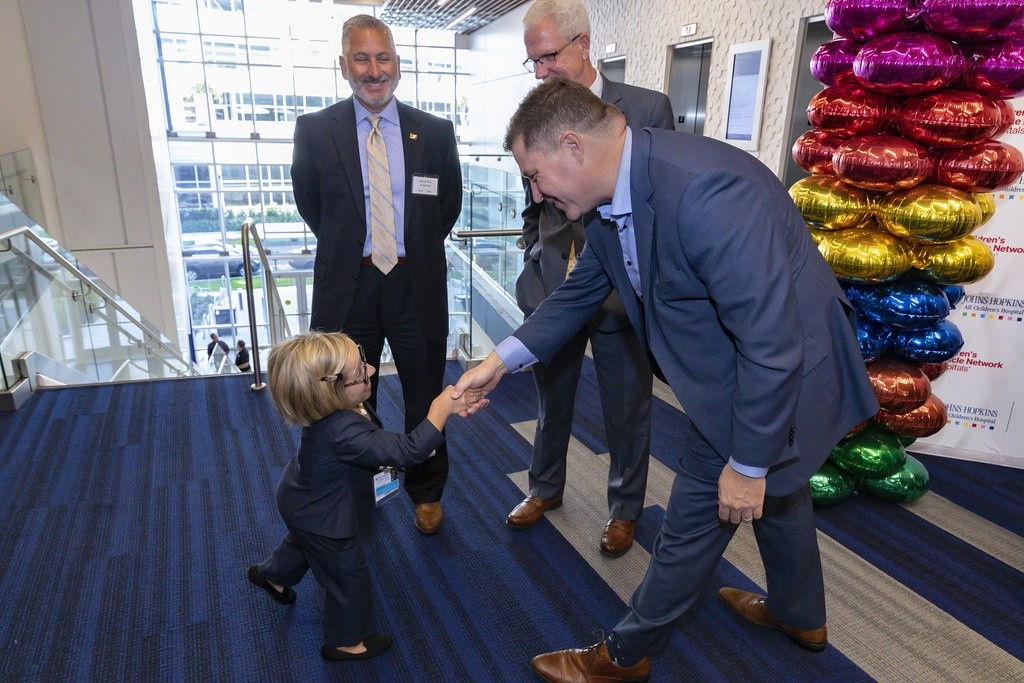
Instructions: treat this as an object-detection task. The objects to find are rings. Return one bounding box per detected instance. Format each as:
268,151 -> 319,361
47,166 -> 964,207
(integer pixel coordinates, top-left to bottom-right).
742,517 -> 752,522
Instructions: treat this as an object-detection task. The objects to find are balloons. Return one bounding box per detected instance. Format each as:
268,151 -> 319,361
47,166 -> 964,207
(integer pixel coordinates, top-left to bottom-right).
787,0 -> 1024,508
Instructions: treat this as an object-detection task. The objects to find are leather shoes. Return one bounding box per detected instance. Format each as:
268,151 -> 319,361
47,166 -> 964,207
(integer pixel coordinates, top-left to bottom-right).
530,629 -> 650,683
413,501 -> 443,533
718,588 -> 827,653
506,494 -> 563,529
599,517 -> 635,556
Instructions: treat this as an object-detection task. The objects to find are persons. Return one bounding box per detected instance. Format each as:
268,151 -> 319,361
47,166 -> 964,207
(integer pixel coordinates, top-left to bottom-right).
450,75 -> 881,683
505,0 -> 675,558
235,340 -> 251,373
290,13 -> 463,532
207,333 -> 229,361
248,334 -> 488,662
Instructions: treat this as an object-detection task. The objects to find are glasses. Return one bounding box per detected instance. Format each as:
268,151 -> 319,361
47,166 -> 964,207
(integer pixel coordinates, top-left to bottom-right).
522,33 -> 581,73
343,344 -> 370,388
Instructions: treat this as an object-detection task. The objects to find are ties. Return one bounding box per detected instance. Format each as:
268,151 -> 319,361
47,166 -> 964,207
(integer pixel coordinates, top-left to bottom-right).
562,241 -> 577,281
366,114 -> 399,276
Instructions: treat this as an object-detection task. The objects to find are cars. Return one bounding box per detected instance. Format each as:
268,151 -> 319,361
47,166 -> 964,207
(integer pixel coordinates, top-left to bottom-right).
288,245 -> 317,269
445,237 -> 512,271
184,240 -> 260,280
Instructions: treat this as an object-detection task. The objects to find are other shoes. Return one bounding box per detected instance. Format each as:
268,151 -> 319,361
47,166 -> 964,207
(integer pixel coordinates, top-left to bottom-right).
247,565 -> 296,605
320,634 -> 392,662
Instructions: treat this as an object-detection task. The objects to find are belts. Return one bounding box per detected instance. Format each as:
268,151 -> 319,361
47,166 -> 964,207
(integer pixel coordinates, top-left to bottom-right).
363,255 -> 407,264
